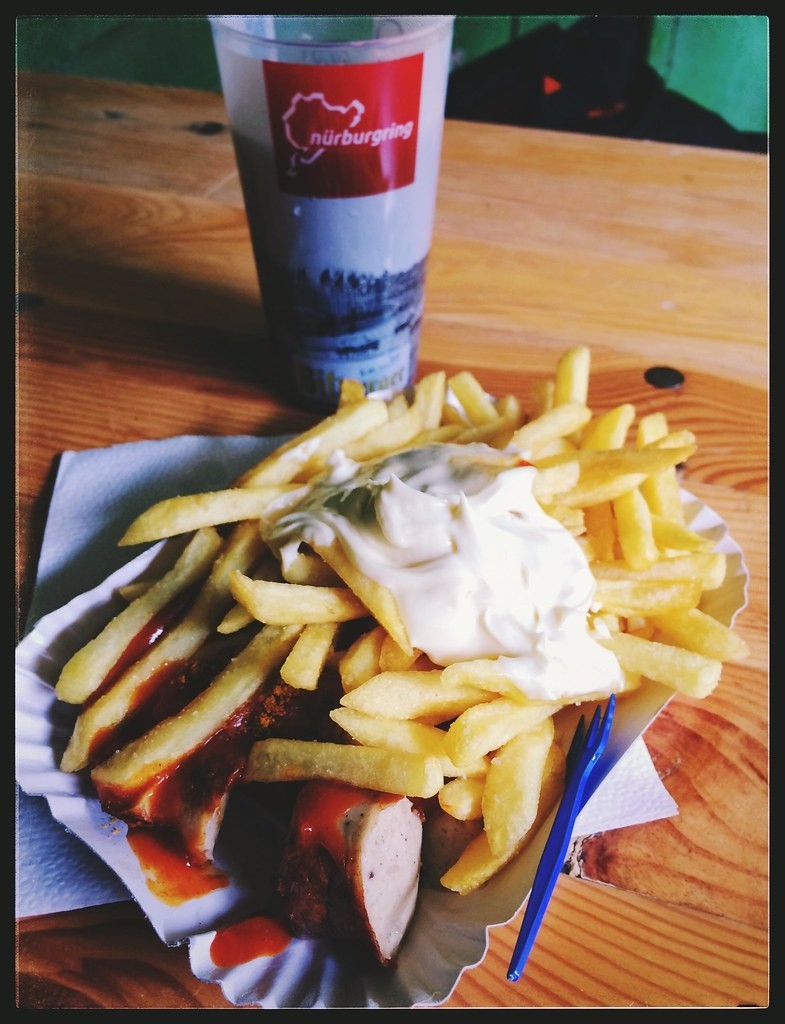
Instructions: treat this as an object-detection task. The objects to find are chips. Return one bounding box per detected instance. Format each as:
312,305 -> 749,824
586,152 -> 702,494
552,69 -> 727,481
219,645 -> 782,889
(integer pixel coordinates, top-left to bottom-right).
56,346 -> 748,898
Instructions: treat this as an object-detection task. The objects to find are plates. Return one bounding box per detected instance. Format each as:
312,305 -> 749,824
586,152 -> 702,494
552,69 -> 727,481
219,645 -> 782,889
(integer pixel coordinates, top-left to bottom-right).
14,375 -> 751,1010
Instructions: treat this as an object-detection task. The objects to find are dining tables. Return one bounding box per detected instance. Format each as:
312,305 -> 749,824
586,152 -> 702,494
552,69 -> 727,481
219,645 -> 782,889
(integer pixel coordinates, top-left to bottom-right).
13,63 -> 771,1008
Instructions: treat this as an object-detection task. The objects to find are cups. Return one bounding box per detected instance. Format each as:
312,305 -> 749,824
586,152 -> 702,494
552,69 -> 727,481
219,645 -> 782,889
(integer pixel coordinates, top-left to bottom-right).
208,14 -> 454,413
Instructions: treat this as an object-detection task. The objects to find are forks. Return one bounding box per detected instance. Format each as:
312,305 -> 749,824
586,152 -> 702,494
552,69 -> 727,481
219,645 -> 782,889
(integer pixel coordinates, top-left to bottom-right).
507,692 -> 614,980
